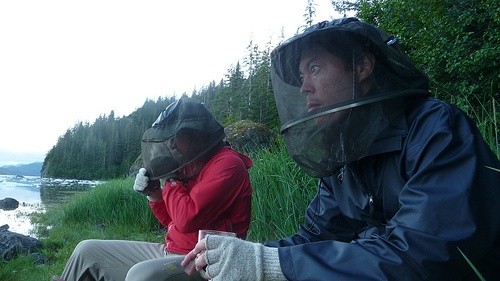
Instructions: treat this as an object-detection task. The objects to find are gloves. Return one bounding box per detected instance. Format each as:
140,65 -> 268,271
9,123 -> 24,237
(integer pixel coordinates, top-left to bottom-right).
151,142 -> 180,190
132,168 -> 161,197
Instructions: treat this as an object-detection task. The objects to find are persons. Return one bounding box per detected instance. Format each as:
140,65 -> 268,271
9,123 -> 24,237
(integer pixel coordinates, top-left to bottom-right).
57,94 -> 251,281
181,14 -> 500,281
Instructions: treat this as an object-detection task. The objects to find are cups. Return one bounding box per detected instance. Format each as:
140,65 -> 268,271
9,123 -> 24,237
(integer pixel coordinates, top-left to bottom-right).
198,230 -> 237,281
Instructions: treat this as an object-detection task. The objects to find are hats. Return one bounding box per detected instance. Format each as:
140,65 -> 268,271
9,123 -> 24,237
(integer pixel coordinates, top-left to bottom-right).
142,99 -> 222,143
271,18 -> 388,87
